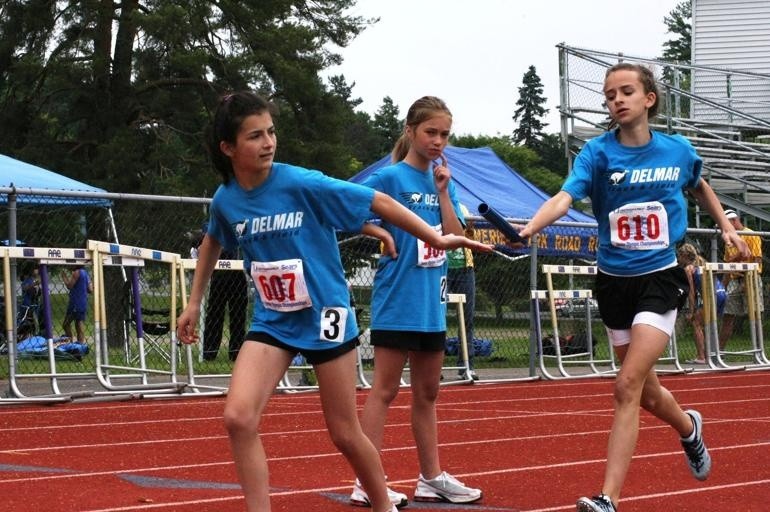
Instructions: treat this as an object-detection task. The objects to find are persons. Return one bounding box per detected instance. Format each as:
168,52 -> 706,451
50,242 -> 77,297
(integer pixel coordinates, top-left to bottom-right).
506,63 -> 751,512
340,95 -> 484,506
439,194 -> 481,382
22,269 -> 41,306
713,209 -> 764,358
198,211 -> 250,361
62,266 -> 93,346
675,243 -> 726,364
176,92 -> 495,512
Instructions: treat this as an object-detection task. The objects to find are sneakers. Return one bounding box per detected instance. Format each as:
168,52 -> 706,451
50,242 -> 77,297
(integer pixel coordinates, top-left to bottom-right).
575,492 -> 619,512
678,408 -> 712,480
350,477 -> 409,507
457,369 -> 480,380
412,470 -> 483,503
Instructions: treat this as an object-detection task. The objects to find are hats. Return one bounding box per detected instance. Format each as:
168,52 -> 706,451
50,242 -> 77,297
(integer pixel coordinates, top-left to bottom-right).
724,209 -> 739,219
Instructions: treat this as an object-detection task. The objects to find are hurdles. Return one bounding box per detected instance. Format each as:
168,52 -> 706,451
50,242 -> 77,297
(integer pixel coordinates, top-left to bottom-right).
343,265 -> 378,391
440,294 -> 475,386
179,257 -> 287,395
87,238 -> 185,399
0,245 -> 107,406
530,264 -> 620,381
655,262 -> 770,377
39,255 -> 148,406
